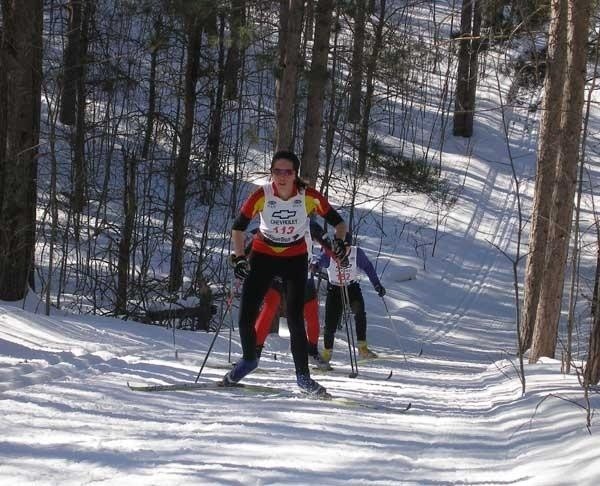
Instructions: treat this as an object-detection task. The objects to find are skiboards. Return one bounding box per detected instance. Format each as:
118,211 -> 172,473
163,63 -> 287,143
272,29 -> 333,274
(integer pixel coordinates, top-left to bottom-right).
126,380 -> 411,413
194,364 -> 393,381
274,348 -> 423,360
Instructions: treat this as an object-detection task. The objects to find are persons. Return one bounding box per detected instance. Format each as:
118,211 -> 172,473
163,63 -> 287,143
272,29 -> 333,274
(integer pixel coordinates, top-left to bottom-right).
227,221 -> 349,370
312,232 -> 386,362
222,150 -> 346,397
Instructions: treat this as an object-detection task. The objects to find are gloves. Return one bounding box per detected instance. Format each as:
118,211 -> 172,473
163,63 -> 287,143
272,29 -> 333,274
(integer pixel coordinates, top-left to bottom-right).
310,260 -> 319,273
235,255 -> 249,280
375,285 -> 386,297
333,238 -> 346,258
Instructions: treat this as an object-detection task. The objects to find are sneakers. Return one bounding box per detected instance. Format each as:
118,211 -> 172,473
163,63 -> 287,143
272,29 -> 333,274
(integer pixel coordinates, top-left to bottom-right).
307,352 -> 330,369
321,347 -> 332,361
297,371 -> 326,396
223,357 -> 258,387
358,346 -> 377,357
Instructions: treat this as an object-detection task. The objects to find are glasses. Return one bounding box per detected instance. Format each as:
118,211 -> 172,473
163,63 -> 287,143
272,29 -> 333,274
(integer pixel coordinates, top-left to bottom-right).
271,167 -> 295,176
